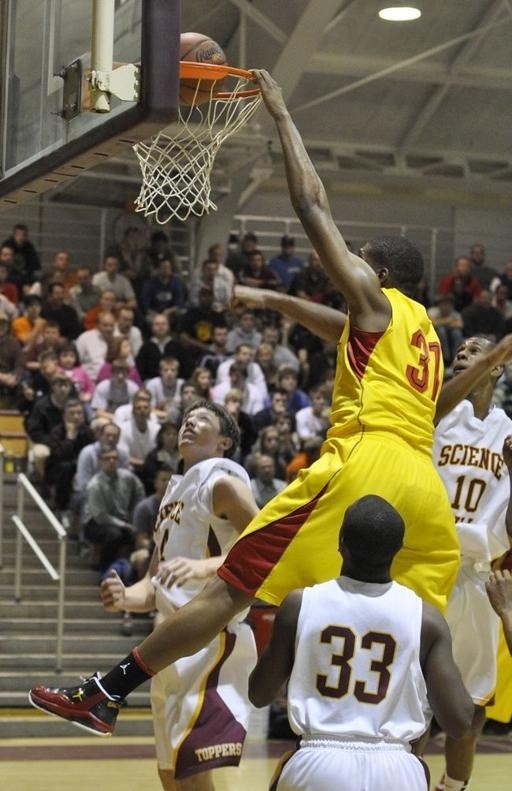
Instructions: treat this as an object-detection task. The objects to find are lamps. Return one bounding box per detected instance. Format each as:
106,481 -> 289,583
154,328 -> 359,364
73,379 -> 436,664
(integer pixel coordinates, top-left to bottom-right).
377,5 -> 423,23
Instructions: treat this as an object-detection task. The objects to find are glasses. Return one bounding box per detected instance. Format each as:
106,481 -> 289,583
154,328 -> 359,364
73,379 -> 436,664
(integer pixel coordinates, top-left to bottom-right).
101,455 -> 118,462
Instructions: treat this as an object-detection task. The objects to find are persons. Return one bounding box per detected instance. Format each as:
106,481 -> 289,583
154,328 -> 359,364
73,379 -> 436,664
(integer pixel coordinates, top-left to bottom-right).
0,262 -> 18,301
434,333 -> 511,741
179,284 -> 217,334
258,425 -> 278,454
102,400 -> 260,791
29,374 -> 75,443
247,453 -> 289,506
224,391 -> 253,453
142,417 -> 184,488
43,401 -> 92,501
42,250 -> 77,296
71,314 -> 115,362
427,245 -> 512,363
89,359 -> 136,414
76,422 -> 129,493
167,256 -> 186,308
29,269 -> 61,298
113,308 -> 142,363
267,371 -> 314,413
29,71 -> 458,739
485,566 -> 511,656
70,267 -> 100,311
143,229 -> 178,273
87,291 -> 118,328
0,226 -> 38,267
132,463 -> 172,585
142,358 -> 185,407
208,365 -> 263,415
255,388 -> 286,427
136,311 -> 192,374
108,225 -> 147,279
165,380 -> 197,423
192,368 -> 216,401
0,314 -> 29,411
93,342 -> 141,382
121,403 -> 159,460
24,322 -> 60,369
112,385 -> 164,424
14,294 -> 44,343
257,343 -> 284,388
48,343 -> 91,393
215,343 -> 266,392
41,282 -> 80,338
259,323 -> 300,371
274,414 -> 301,461
93,256 -> 137,307
197,324 -> 228,365
226,311 -> 263,350
296,386 -> 341,443
203,233 -> 354,308
85,443 -> 144,570
298,333 -> 321,368
0,292 -> 19,321
0,245 -> 33,285
250,493 -> 475,791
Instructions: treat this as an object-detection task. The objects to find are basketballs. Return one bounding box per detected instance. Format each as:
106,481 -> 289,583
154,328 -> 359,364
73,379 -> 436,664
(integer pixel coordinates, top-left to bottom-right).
180,32 -> 227,107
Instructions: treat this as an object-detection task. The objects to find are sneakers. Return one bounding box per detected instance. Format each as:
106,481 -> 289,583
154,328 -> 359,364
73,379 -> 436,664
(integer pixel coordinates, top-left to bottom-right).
29,672 -> 120,737
122,618 -> 136,636
